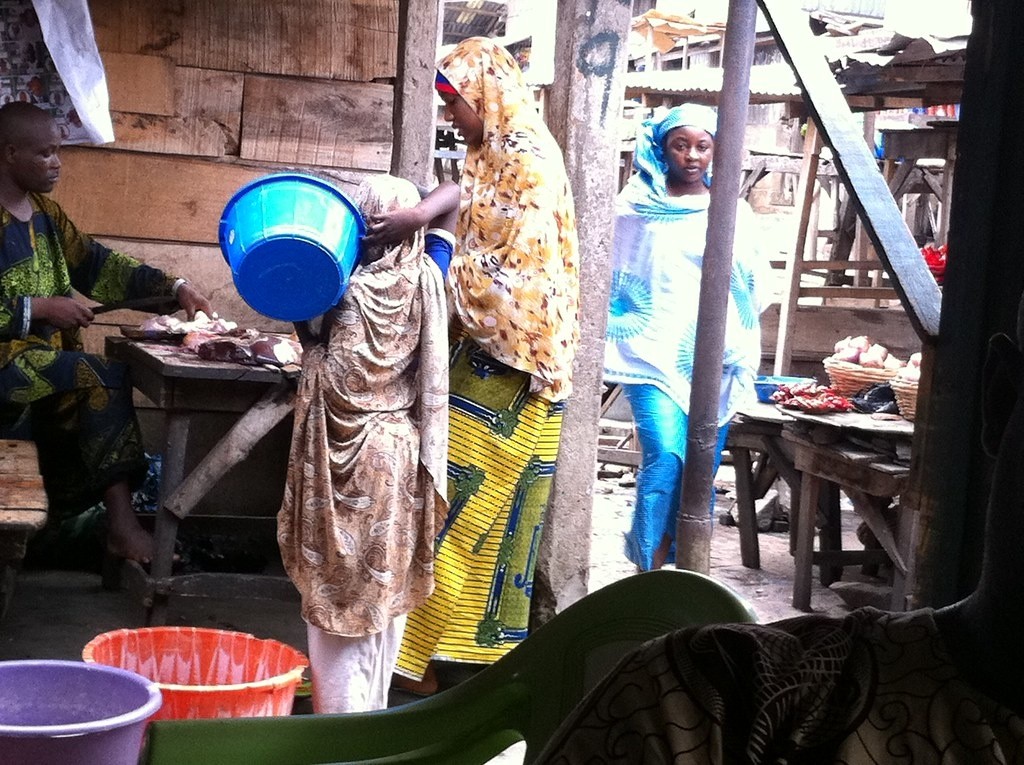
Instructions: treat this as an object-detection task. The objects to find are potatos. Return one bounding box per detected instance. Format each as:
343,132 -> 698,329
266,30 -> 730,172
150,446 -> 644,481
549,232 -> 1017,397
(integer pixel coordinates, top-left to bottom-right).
899,352 -> 921,383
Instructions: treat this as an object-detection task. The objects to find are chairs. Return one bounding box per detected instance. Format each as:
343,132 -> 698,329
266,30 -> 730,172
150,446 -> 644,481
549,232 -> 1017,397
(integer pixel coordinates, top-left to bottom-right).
141,569 -> 754,765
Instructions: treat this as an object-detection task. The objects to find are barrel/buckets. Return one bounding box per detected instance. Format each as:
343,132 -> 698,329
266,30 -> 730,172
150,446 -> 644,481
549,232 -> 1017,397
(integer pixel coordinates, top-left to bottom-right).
0,659 -> 162,765
217,172 -> 366,323
81,626 -> 310,723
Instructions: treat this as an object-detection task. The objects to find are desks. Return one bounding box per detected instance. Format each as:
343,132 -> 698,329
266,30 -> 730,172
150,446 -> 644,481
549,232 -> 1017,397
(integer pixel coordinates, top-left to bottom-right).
104,336 -> 304,627
0,440 -> 47,625
849,119 -> 959,308
723,399 -> 911,611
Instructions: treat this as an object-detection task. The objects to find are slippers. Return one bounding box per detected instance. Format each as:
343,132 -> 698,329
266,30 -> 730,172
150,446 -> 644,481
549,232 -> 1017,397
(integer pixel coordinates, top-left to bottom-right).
389,679 -> 432,697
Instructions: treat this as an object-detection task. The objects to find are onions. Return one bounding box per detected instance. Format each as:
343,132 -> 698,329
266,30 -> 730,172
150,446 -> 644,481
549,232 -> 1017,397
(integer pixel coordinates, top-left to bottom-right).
831,333 -> 889,369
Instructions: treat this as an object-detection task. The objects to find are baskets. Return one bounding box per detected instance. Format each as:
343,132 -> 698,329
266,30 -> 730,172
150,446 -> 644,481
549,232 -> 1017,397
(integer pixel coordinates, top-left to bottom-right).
822,355 -> 895,397
890,374 -> 920,420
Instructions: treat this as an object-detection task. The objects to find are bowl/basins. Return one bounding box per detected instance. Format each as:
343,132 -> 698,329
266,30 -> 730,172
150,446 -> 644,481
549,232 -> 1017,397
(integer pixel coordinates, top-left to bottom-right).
754,375 -> 813,404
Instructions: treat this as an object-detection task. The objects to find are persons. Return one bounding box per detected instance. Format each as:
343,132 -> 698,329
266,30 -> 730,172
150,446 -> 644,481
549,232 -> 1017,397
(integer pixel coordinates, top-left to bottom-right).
0,101 -> 220,563
603,102 -> 773,574
392,36 -> 579,701
276,175 -> 461,714
